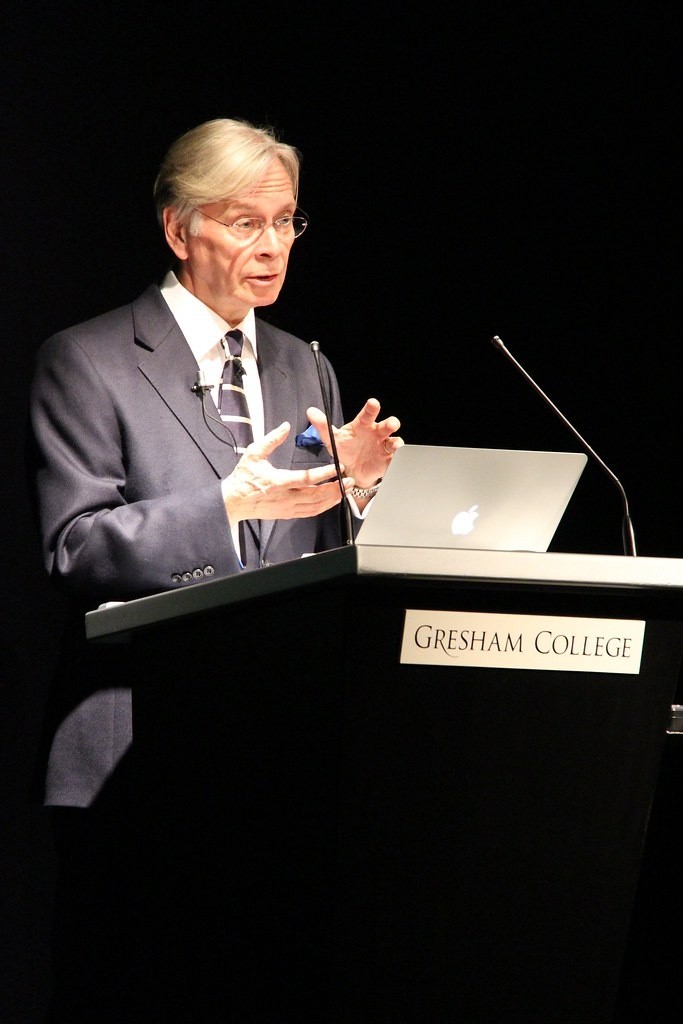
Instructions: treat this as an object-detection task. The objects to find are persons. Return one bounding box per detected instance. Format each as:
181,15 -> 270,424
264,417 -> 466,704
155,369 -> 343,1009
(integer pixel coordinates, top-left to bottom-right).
28,120 -> 400,1022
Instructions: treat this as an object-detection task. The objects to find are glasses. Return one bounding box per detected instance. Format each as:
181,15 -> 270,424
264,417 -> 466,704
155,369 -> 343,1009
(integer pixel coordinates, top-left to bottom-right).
179,197 -> 308,243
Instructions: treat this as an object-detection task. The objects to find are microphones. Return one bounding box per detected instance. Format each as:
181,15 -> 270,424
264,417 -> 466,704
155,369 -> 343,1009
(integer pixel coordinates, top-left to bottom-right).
489,335 -> 638,557
310,341 -> 355,547
196,371 -> 206,394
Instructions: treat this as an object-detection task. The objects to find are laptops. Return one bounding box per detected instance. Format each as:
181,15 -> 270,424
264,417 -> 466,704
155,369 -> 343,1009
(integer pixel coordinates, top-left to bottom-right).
357,444 -> 589,553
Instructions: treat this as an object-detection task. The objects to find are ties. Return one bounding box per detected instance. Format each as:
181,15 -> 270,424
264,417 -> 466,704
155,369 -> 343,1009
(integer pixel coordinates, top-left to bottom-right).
217,332 -> 253,459
